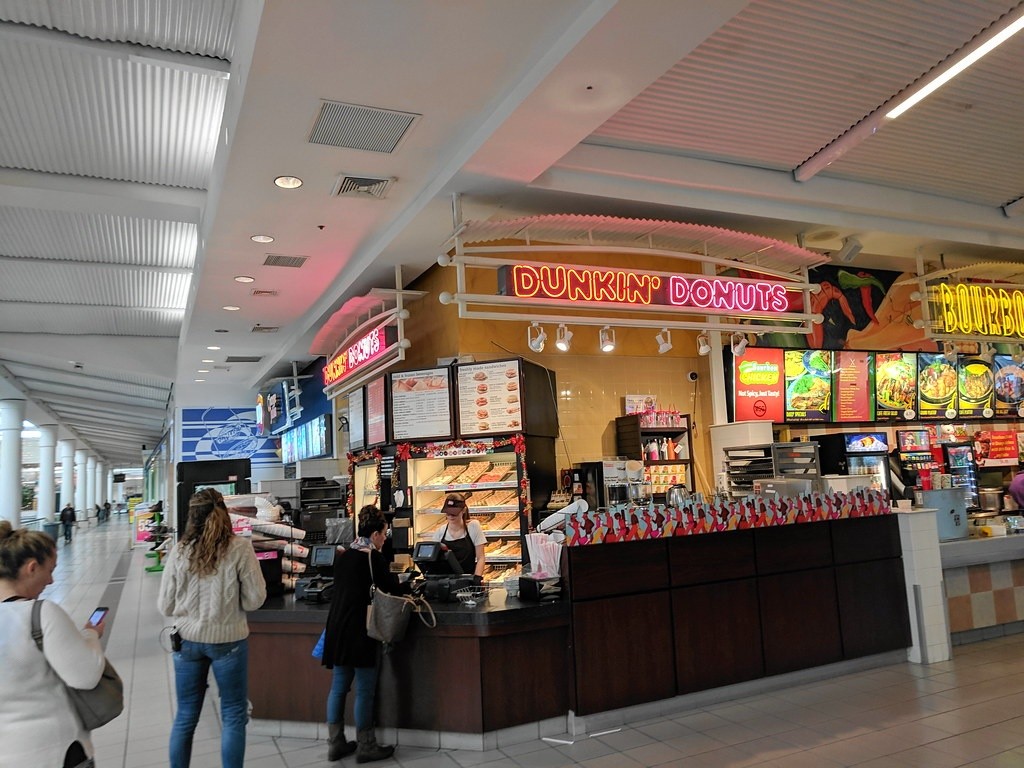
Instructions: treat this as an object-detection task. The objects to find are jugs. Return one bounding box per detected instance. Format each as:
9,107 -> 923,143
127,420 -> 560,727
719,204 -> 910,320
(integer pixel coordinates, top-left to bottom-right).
667,484 -> 691,507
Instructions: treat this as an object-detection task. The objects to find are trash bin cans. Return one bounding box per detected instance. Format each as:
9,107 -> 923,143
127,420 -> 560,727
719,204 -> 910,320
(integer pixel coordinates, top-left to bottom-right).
42,522 -> 60,542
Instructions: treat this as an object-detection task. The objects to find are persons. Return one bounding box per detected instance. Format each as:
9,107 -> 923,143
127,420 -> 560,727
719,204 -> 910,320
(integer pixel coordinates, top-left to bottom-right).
321,505 -> 414,763
432,493 -> 487,586
157,488 -> 267,768
96,501 -> 110,521
1008,462 -> 1024,507
60,504 -> 76,542
0,520 -> 105,768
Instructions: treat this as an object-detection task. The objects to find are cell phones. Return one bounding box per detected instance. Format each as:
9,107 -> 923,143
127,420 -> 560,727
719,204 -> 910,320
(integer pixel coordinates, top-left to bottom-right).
88,606 -> 110,627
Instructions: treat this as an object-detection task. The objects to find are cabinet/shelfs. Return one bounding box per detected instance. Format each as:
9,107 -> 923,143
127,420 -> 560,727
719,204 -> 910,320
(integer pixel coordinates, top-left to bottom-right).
411,454 -> 522,587
722,441 -> 822,499
615,413 -> 696,503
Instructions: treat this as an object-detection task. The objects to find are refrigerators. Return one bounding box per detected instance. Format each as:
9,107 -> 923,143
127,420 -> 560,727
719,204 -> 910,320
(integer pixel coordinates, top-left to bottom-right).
809,432 -> 892,500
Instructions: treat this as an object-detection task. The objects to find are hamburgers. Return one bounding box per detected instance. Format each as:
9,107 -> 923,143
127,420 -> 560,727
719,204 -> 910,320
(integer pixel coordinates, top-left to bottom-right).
475,368 -> 519,430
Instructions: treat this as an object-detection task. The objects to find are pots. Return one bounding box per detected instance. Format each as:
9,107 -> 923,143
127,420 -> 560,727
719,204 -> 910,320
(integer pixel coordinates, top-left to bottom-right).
979,485 -> 1004,511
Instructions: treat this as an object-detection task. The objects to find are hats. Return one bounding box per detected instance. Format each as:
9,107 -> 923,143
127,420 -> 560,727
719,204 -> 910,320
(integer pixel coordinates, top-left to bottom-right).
442,494 -> 465,517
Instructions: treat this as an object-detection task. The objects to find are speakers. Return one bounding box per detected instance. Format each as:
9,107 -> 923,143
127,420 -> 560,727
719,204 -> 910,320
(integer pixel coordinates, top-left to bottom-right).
839,239 -> 863,263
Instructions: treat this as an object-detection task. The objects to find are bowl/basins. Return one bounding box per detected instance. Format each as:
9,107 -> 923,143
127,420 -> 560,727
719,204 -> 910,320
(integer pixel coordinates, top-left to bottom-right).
919,359 -> 1023,402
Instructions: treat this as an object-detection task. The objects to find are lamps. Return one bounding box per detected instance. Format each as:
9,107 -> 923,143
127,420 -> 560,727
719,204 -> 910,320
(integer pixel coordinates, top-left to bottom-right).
599,326 -> 615,352
555,324 -> 573,352
731,332 -> 748,356
696,331 -> 711,356
527,321 -> 547,353
794,6 -> 1024,181
655,328 -> 672,354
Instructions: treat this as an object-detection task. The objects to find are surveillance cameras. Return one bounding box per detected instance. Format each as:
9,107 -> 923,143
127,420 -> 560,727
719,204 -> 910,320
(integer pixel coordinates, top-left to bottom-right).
687,371 -> 698,383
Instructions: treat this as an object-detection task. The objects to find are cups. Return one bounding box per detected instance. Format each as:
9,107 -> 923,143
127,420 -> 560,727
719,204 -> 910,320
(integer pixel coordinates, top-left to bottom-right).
618,461 -> 643,481
251,535 -> 309,587
537,499 -> 589,533
228,513 -> 306,539
626,410 -> 681,428
918,468 -> 952,490
890,500 -> 912,512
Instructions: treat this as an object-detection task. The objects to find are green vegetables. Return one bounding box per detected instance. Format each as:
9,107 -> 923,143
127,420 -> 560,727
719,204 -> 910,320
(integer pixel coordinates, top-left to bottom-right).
794,356 -> 829,393
929,359 -> 944,374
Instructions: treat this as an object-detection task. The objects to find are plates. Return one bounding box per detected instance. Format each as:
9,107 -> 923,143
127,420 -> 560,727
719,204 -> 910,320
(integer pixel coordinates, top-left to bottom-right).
920,395 -> 1024,419
875,361 -> 916,419
784,350 -> 830,410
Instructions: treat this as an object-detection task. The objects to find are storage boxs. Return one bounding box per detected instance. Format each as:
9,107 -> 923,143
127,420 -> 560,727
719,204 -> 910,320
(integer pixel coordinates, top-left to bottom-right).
824,473 -> 871,494
752,479 -> 813,500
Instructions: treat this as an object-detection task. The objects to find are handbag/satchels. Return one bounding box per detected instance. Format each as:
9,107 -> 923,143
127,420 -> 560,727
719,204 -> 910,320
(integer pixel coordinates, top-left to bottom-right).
30,597 -> 125,731
367,549 -> 437,646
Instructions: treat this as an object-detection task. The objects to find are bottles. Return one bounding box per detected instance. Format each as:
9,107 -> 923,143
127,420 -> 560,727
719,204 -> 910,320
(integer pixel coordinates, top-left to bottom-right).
642,437 -> 675,461
847,458 -> 885,493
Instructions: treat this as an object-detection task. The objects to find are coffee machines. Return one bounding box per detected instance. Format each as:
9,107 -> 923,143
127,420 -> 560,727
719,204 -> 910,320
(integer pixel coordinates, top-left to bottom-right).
609,481 -> 665,511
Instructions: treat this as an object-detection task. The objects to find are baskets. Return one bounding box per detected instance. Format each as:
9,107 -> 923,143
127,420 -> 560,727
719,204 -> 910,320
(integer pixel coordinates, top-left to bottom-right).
452,586 -> 495,604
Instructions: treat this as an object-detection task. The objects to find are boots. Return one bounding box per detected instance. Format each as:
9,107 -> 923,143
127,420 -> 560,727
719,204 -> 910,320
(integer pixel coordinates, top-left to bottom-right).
326,722 -> 358,761
354,728 -> 395,763
